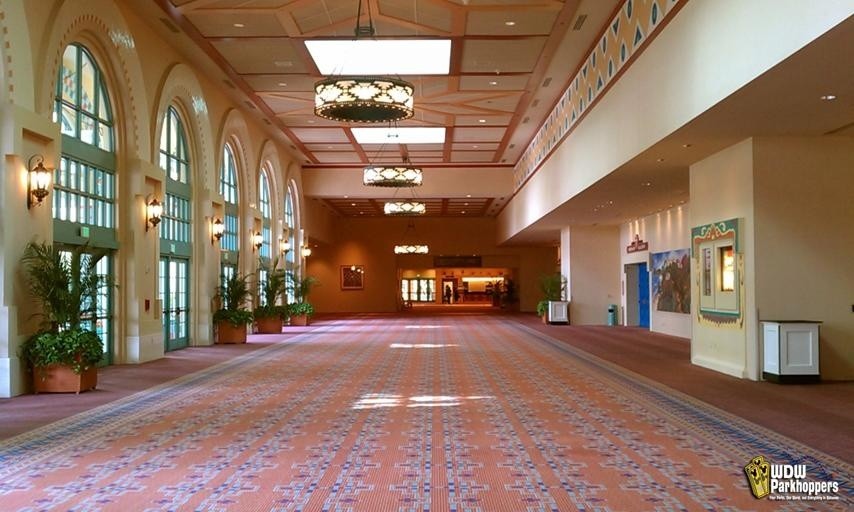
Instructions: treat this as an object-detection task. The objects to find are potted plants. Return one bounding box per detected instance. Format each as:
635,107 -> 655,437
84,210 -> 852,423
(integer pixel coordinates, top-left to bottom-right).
251,256 -> 290,333
484,279 -> 502,307
215,266 -> 259,345
281,264 -> 319,325
16,235 -> 121,397
536,274 -> 570,325
500,276 -> 519,312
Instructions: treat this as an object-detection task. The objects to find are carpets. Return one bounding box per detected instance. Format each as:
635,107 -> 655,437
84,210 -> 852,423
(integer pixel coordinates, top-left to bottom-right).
0,318 -> 854,511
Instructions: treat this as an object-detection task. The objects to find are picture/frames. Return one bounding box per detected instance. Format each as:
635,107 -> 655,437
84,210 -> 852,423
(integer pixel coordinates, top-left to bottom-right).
340,264 -> 365,290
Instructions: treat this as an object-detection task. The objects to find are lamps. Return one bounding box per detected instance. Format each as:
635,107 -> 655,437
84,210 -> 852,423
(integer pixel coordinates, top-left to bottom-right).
315,0 -> 414,123
278,234 -> 289,257
143,189 -> 164,231
364,121 -> 422,188
208,214 -> 224,247
383,186 -> 425,216
303,245 -> 311,263
393,214 -> 429,257
25,151 -> 51,208
252,228 -> 262,252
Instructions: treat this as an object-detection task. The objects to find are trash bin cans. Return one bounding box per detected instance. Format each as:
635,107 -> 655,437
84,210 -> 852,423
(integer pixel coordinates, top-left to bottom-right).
608,304 -> 618,326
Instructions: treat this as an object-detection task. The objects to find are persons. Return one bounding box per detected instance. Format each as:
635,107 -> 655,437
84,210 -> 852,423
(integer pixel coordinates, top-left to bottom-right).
445,285 -> 452,304
